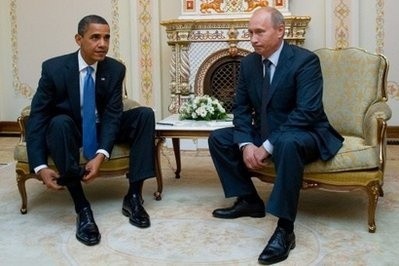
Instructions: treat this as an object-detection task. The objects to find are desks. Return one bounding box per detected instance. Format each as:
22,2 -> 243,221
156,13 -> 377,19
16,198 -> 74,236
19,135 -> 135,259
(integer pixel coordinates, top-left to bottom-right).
153,114 -> 234,201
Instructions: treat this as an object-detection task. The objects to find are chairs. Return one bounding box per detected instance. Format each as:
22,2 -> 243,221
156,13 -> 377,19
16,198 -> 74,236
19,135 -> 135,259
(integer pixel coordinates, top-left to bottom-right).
13,60 -> 167,213
248,47 -> 393,233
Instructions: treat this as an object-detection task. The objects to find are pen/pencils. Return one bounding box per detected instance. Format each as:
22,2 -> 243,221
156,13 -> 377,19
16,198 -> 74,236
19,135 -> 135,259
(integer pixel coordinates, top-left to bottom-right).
157,122 -> 173,126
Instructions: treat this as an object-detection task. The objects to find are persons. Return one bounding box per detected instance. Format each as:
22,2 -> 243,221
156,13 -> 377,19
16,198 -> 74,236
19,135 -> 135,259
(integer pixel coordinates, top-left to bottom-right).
25,15 -> 157,245
208,7 -> 344,264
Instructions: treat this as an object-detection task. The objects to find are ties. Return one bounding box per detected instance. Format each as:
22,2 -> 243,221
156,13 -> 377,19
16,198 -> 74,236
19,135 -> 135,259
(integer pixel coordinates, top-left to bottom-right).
260,59 -> 273,142
83,66 -> 99,160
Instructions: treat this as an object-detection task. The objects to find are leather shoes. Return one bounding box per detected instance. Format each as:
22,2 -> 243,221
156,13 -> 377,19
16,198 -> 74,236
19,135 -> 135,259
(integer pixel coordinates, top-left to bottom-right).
258,226 -> 295,265
212,198 -> 266,218
122,192 -> 151,228
75,207 -> 101,246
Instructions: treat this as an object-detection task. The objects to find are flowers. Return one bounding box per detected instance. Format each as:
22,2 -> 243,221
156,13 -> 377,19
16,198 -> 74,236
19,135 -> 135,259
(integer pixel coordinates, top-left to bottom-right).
178,95 -> 228,121
